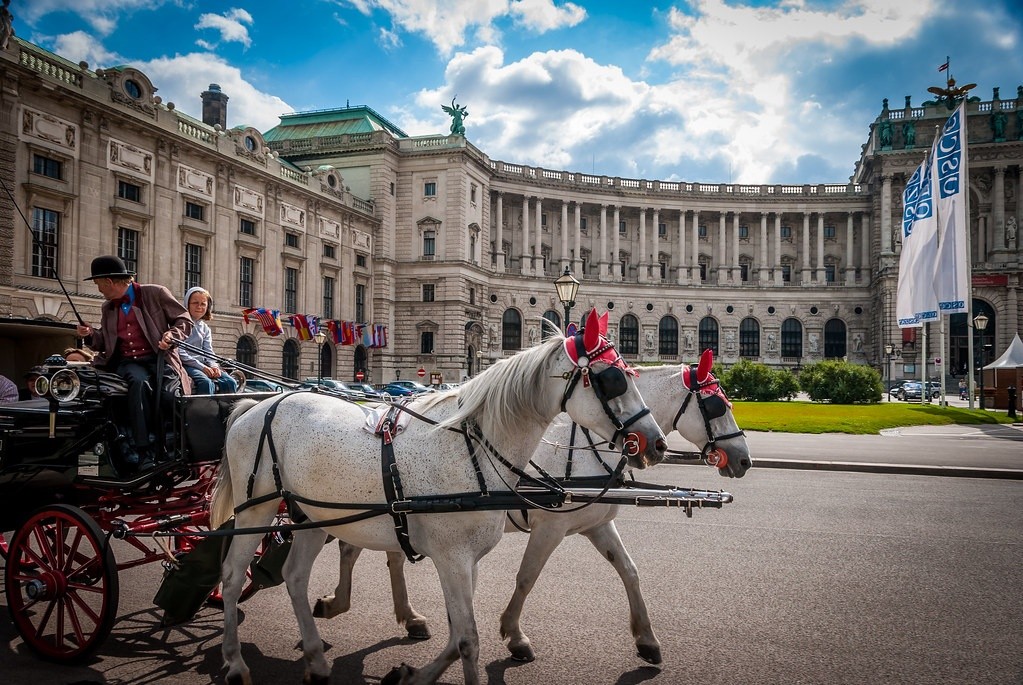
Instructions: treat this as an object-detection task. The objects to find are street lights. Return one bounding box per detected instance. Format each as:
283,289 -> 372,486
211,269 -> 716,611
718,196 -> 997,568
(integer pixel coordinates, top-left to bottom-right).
552,266 -> 580,332
885,344 -> 894,402
974,309 -> 990,410
314,330 -> 326,388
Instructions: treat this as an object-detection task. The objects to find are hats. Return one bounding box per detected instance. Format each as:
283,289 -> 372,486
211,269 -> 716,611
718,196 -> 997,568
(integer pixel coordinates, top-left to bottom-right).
82,255 -> 137,281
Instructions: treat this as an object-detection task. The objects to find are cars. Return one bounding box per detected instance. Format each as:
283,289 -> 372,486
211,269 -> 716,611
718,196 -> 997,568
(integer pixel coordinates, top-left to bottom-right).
298,377 -> 461,404
889,378 -> 942,401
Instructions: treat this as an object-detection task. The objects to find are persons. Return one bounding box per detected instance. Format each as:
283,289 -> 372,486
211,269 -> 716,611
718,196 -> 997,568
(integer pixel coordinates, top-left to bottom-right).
172,286 -> 238,394
1006,215 -> 1017,239
903,116 -> 920,145
491,324 -> 498,342
852,333 -> 864,352
810,334 -> 818,352
951,361 -> 980,401
768,332 -> 775,351
530,325 -> 537,344
685,330 -> 695,348
726,331 -> 735,349
647,331 -> 654,348
1016,110 -> 1023,140
0,347 -> 95,404
894,221 -> 901,243
608,330 -> 616,343
78,255 -> 193,470
882,119 -> 890,145
452,97 -> 465,134
994,109 -> 1007,137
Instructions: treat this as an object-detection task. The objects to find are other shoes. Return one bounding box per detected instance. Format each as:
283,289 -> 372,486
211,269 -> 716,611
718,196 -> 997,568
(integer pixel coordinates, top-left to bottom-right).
154,452 -> 173,465
139,459 -> 157,474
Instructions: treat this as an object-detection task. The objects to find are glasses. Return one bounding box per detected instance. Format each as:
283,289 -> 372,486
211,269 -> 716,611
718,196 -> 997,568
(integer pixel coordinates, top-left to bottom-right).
189,302 -> 207,307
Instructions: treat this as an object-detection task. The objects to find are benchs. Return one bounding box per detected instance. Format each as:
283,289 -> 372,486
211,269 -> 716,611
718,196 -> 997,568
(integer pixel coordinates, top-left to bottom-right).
72,361 -> 240,402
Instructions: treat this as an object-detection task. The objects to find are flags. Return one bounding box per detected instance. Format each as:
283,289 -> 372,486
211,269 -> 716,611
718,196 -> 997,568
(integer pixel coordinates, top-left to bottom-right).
938,63 -> 948,72
243,308 -> 387,348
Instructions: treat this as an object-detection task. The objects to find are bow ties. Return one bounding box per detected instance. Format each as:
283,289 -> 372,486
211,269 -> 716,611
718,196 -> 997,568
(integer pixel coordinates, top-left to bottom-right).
111,295 -> 129,308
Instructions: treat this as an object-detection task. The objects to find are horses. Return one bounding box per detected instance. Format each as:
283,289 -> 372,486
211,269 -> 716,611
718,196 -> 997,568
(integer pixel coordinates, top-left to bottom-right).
310,346 -> 751,663
210,304 -> 669,685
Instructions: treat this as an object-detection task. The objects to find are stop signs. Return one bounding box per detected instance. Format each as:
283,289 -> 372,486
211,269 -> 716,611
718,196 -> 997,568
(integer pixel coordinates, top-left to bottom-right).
356,371 -> 364,380
417,368 -> 425,377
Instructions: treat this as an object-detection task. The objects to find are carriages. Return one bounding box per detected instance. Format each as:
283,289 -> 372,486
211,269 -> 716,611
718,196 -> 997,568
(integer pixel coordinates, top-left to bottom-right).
0,305 -> 755,685
